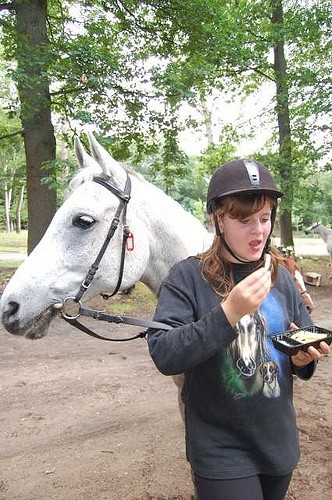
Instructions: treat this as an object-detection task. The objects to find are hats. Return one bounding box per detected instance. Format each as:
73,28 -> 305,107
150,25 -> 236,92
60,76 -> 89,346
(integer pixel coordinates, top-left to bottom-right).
206,158 -> 285,202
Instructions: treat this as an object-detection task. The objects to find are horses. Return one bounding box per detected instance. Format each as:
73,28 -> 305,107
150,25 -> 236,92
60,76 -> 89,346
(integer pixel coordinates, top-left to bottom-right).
0,131 -> 332,424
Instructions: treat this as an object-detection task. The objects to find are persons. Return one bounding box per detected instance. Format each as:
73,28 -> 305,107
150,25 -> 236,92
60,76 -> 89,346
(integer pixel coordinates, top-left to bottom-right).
148,159 -> 332,500
